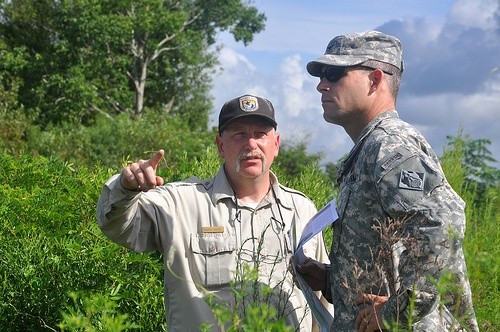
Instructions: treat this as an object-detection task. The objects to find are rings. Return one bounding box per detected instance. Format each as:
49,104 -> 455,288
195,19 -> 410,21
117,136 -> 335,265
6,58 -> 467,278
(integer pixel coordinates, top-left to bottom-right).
364,319 -> 369,323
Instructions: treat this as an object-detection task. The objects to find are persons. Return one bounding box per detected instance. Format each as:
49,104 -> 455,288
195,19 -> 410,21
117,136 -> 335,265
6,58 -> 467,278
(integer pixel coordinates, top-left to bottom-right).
288,30 -> 479,332
97,95 -> 334,332
